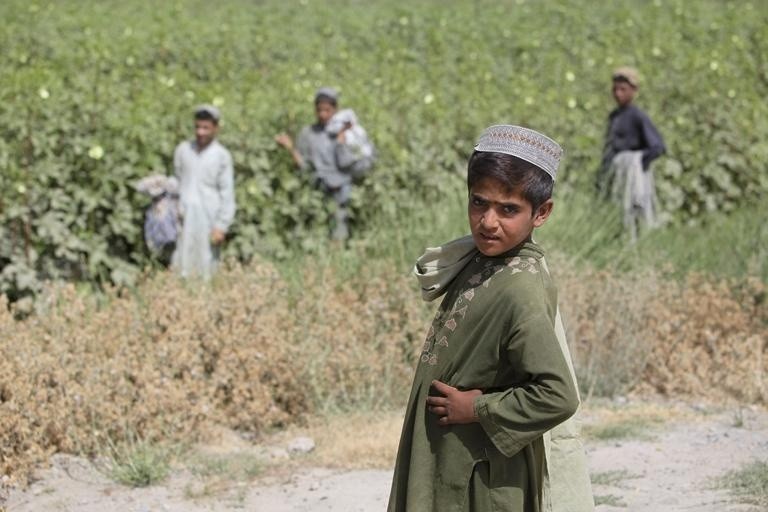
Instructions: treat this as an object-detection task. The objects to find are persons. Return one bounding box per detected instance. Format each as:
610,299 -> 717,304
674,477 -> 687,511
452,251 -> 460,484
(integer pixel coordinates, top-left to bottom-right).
387,124 -> 594,512
277,87 -> 375,246
168,103 -> 235,278
600,65 -> 666,242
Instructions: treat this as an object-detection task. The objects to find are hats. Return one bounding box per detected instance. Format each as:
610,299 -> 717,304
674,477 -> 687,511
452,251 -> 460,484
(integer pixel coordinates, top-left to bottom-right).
610,66 -> 641,88
193,104 -> 220,123
474,124 -> 565,183
315,87 -> 339,104
